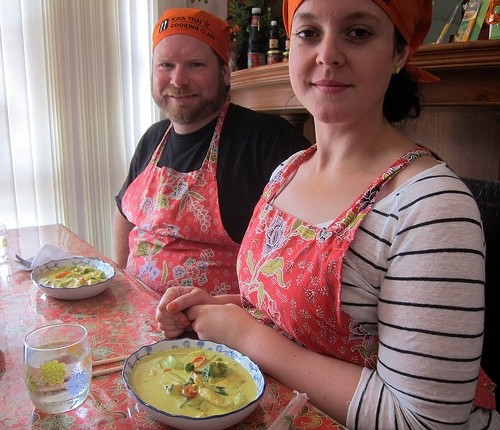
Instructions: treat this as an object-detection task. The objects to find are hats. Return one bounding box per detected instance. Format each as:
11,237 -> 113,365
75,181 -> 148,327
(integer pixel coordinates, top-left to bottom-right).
283,0 -> 442,83
153,8 -> 232,64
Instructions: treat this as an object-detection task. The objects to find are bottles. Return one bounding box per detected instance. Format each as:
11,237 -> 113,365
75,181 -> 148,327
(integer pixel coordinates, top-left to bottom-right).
267,21 -> 280,65
283,39 -> 289,63
247,8 -> 264,68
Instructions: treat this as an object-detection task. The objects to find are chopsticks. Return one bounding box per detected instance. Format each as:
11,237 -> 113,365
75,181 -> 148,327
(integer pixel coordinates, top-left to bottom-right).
91,355 -> 128,378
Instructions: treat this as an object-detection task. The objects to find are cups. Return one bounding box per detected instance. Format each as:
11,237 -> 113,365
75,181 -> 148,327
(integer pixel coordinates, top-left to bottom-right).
0,223 -> 8,263
22,323 -> 93,414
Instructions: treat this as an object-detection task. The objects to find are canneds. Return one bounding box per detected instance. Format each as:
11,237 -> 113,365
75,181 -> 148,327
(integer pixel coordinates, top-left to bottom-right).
267,50 -> 289,64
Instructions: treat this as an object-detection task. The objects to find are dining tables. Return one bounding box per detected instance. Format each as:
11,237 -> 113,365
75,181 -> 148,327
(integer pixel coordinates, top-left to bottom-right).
0,223 -> 350,430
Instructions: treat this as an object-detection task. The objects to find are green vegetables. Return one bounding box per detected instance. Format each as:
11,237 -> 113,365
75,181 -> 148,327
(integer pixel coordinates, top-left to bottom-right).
165,357 -> 228,408
45,267 -> 105,287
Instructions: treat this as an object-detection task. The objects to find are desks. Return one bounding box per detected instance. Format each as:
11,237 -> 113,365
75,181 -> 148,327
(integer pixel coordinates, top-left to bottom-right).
228,38 -> 500,137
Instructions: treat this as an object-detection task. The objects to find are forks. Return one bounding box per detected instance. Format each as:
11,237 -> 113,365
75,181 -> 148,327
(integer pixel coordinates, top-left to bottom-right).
15,255 -> 32,268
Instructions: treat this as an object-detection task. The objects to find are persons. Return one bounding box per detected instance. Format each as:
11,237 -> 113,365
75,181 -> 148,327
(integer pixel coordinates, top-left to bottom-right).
113,8 -> 310,299
156,0 -> 500,430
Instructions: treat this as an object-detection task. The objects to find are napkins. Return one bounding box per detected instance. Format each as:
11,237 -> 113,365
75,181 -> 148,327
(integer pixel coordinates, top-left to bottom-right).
10,244 -> 84,272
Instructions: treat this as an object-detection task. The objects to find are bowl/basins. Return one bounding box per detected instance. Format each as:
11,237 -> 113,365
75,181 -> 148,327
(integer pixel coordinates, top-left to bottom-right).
29,256 -> 116,300
121,338 -> 266,430
